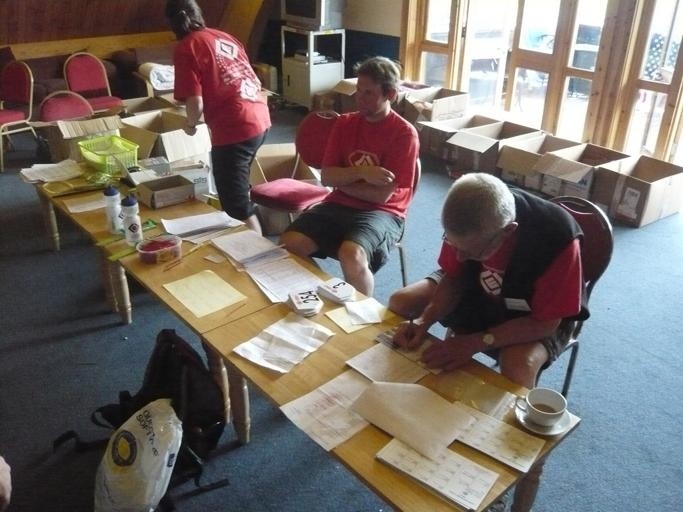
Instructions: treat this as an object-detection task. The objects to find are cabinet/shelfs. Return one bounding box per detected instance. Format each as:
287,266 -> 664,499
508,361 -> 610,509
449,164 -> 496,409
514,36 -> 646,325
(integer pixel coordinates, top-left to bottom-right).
280,24 -> 346,112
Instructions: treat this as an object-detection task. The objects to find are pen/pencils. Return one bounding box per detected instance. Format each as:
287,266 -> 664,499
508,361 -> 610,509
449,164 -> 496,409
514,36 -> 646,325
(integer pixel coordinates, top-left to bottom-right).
407,307 -> 414,343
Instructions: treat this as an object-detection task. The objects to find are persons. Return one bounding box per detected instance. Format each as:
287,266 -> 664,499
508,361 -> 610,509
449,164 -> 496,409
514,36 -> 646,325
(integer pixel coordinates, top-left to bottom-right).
279,55 -> 420,297
389,171 -> 581,390
167,0 -> 273,238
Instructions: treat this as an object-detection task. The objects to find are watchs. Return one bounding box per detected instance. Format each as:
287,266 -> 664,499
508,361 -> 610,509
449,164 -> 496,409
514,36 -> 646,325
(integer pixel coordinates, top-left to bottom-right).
480,330 -> 499,355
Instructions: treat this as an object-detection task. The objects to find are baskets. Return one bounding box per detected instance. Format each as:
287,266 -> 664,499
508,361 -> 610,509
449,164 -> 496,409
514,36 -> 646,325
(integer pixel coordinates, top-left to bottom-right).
77,134 -> 140,174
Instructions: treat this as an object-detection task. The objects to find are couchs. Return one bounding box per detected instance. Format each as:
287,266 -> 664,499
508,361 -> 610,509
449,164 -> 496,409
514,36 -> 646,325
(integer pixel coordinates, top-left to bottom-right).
21,53 -> 124,140
114,43 -> 177,96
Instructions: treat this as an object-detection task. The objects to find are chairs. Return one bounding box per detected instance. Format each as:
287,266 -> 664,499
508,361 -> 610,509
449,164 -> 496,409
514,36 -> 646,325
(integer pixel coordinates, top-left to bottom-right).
446,196 -> 615,398
0,60 -> 39,173
393,157 -> 422,288
249,109 -> 340,224
39,90 -> 94,121
63,51 -> 128,117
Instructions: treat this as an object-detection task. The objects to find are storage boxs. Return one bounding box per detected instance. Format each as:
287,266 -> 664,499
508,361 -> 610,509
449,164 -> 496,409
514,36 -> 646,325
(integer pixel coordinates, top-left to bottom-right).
25,104 -> 128,161
119,96 -> 175,116
25,112 -> 129,162
171,164 -> 209,196
248,143 -> 322,236
136,175 -> 195,209
117,106 -> 212,166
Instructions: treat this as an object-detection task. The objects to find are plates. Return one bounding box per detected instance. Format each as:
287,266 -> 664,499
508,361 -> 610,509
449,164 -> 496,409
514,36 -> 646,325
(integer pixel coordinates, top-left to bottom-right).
515,397 -> 571,436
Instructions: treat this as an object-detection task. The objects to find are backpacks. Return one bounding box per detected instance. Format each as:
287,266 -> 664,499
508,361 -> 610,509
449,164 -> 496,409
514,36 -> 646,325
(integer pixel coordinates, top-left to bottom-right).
92,329 -> 227,479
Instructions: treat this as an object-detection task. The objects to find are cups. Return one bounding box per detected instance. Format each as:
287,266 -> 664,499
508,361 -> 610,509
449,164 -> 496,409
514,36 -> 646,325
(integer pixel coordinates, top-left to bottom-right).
515,387 -> 568,427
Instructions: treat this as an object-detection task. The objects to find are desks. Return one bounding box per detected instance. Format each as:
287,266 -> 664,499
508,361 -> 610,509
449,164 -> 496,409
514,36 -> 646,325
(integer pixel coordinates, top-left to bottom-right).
31,160 -> 216,313
201,281 -> 581,512
104,210 -> 335,425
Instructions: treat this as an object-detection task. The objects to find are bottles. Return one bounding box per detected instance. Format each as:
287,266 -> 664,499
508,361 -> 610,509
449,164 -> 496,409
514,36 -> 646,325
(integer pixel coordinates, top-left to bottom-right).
121,195 -> 143,248
103,185 -> 122,234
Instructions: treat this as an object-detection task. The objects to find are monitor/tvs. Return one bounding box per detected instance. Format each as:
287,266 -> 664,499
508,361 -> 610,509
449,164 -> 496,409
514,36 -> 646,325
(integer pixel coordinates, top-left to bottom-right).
281,0 -> 344,31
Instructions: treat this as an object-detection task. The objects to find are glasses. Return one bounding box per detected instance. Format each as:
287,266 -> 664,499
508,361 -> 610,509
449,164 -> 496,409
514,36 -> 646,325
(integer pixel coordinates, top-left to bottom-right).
441,230 -> 503,260
